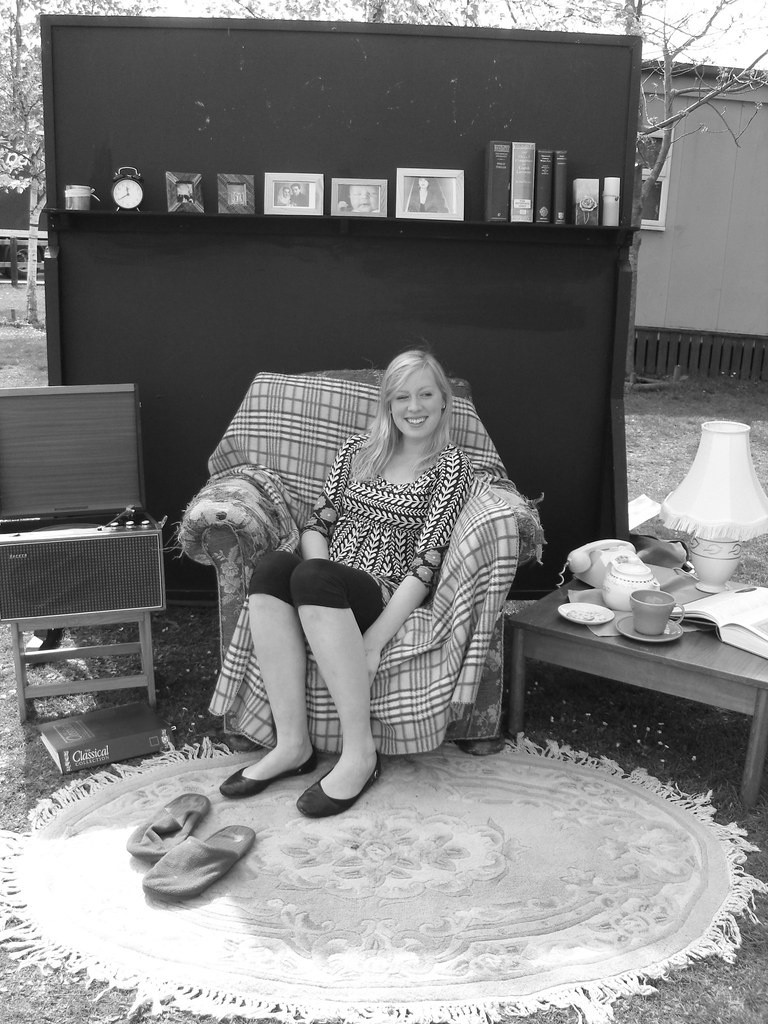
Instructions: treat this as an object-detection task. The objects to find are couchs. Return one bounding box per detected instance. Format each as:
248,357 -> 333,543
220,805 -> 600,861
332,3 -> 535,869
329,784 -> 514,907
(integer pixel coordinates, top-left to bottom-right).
176,370 -> 549,755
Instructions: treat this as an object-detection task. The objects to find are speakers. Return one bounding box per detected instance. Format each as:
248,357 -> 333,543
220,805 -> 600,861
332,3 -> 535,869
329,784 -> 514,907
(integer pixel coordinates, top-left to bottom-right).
0,383 -> 167,622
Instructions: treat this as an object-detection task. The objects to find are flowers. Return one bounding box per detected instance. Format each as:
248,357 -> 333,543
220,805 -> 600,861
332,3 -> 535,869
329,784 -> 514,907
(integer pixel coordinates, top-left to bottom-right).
580,196 -> 598,212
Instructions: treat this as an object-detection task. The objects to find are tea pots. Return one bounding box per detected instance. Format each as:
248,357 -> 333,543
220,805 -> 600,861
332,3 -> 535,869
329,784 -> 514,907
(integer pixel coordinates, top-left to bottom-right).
602,550 -> 661,611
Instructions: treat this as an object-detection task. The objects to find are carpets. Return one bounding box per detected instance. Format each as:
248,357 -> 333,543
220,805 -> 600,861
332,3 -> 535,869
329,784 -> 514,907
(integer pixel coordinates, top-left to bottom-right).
0,731 -> 768,1024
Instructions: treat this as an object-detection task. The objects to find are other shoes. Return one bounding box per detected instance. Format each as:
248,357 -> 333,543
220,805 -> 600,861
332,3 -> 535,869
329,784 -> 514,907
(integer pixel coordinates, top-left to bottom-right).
219,744 -> 317,799
296,749 -> 380,817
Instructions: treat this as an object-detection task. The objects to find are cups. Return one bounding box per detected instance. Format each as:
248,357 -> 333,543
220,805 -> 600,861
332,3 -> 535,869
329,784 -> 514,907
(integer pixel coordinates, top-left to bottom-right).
65,185 -> 90,209
630,589 -> 685,635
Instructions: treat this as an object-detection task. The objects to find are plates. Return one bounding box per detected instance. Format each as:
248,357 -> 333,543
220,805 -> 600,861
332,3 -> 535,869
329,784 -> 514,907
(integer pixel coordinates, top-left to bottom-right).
558,602 -> 615,626
615,615 -> 683,642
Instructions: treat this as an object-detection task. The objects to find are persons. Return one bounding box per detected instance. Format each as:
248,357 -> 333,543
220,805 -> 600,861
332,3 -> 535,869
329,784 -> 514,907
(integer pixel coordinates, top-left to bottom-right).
408,177 -> 439,212
337,186 -> 379,212
276,183 -> 308,207
219,335 -> 475,816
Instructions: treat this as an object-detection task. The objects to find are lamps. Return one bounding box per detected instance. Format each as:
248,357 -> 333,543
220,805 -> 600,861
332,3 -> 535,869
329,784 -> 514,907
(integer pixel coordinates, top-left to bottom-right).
661,421 -> 768,594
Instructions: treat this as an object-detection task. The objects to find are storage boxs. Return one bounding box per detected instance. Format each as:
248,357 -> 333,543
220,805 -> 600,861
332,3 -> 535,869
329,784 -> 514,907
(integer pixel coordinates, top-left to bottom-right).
483,140 -> 534,222
0,383 -> 166,625
572,179 -> 599,225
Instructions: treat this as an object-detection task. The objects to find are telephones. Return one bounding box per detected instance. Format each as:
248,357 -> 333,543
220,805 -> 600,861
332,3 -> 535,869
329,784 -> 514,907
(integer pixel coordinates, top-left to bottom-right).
565,539 -> 636,587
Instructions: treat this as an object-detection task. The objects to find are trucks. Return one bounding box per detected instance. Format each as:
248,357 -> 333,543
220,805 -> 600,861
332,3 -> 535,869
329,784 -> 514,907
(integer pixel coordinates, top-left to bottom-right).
0,184 -> 48,281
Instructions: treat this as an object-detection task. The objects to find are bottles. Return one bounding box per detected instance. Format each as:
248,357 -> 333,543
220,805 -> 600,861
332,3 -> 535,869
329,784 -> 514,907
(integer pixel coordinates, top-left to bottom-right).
602,177 -> 619,227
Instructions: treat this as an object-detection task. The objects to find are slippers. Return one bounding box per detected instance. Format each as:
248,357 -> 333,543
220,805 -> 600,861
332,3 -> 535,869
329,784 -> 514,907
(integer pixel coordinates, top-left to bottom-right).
127,794 -> 210,856
142,825 -> 256,899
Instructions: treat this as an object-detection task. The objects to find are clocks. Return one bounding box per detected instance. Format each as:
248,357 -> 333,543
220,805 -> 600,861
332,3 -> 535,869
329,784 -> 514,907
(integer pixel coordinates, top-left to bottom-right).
111,167 -> 143,212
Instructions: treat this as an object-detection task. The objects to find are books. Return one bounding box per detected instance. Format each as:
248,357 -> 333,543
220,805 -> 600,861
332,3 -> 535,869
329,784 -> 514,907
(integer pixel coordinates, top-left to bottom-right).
668,587 -> 768,661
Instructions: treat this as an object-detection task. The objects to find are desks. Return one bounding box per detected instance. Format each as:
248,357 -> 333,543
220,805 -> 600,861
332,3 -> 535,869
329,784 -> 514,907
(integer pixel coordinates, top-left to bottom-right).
505,562 -> 767,809
10,611 -> 156,724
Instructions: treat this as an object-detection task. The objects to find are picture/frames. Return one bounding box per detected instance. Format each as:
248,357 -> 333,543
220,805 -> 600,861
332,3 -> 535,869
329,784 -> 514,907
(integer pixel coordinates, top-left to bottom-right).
263,172 -> 324,216
330,178 -> 388,218
395,168 -> 464,221
217,173 -> 255,215
166,171 -> 205,213
